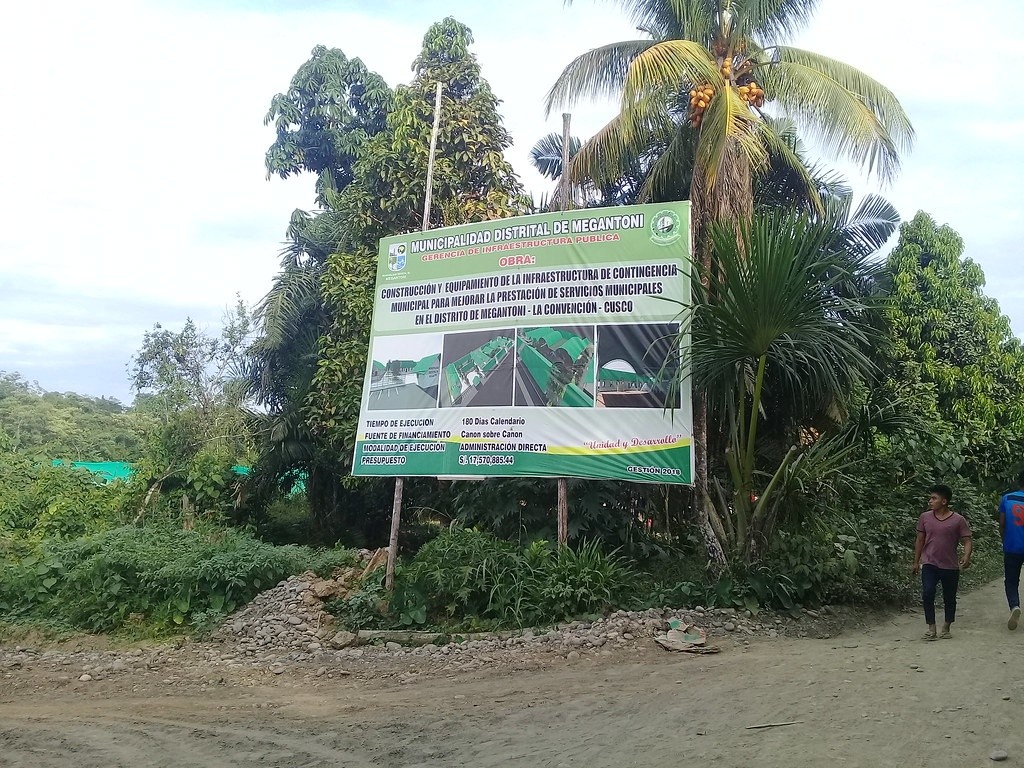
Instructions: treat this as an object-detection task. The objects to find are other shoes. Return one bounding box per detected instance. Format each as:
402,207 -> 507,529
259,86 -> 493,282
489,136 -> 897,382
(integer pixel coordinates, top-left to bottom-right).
922,629 -> 936,640
1008,606 -> 1022,630
939,631 -> 951,639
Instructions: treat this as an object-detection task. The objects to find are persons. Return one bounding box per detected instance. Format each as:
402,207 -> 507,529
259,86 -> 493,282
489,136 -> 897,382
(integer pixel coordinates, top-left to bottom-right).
913,485 -> 972,640
999,472 -> 1024,631
